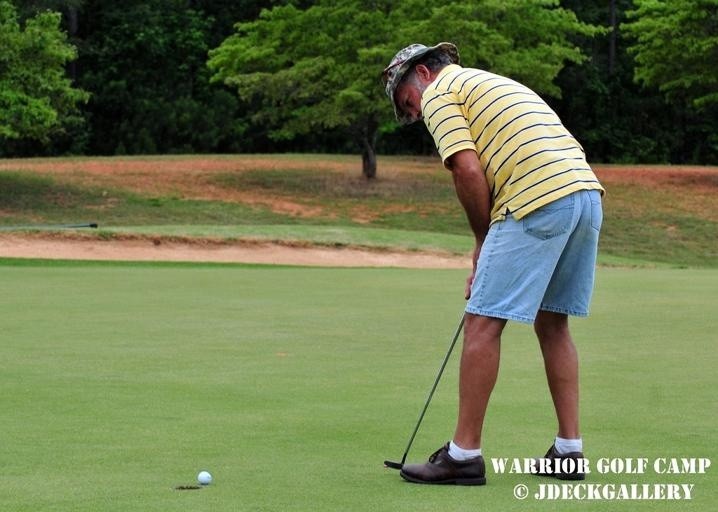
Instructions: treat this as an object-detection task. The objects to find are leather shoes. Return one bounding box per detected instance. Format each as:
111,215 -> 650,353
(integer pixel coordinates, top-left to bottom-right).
529,443 -> 587,480
400,441 -> 488,488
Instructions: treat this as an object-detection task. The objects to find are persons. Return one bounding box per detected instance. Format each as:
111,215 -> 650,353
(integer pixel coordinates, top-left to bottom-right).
383,42 -> 606,487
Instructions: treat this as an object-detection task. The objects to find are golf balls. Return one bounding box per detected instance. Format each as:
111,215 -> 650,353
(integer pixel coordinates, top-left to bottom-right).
198,471 -> 211,484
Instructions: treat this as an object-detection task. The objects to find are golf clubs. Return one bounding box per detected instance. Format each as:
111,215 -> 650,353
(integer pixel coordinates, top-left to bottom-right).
383,311 -> 464,469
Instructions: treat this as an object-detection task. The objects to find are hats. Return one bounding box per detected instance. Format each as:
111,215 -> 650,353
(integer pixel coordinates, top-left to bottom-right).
383,41 -> 461,124
